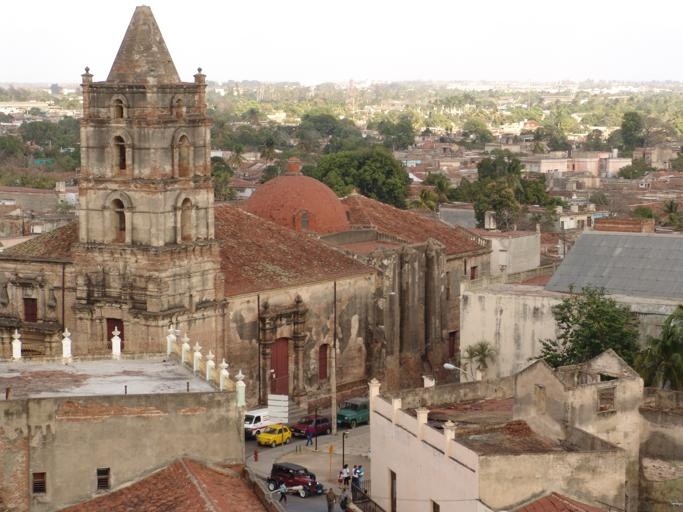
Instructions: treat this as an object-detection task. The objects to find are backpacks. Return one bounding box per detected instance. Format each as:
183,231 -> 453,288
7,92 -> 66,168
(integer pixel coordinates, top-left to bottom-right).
327,494 -> 334,502
340,495 -> 352,510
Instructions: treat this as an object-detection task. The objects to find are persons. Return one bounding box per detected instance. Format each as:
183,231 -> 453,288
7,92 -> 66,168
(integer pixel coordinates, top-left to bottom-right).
327,488 -> 337,512
338,464 -> 364,491
339,488 -> 347,512
306,432 -> 313,446
279,481 -> 288,502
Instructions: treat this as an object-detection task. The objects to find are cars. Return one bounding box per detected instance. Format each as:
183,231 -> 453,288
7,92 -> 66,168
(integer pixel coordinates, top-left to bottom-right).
256,424 -> 292,448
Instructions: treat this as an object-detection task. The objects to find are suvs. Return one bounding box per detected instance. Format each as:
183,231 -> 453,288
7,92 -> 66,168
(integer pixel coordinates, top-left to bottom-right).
337,396 -> 370,429
290,414 -> 332,439
266,462 -> 323,498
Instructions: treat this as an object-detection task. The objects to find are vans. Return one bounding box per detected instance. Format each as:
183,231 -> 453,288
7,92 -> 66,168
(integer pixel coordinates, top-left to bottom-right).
244,408 -> 282,440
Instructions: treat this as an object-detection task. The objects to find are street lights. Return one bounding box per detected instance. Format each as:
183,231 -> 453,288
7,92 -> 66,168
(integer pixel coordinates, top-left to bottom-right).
315,405 -> 322,452
342,431 -> 350,469
